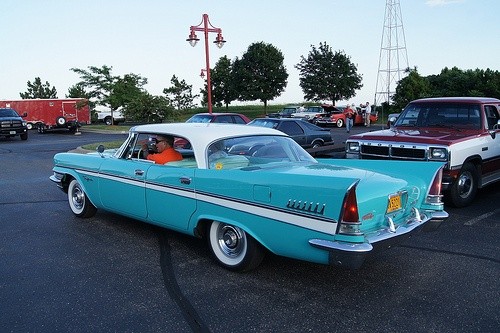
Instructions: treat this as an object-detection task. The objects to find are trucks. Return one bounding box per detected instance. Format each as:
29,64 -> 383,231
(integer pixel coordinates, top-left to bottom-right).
0,98 -> 92,130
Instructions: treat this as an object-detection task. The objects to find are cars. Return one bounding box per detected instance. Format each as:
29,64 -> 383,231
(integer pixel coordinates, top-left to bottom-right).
387,108 -> 420,129
48,121 -> 449,275
266,104 -> 379,128
221,116 -> 335,157
147,112 -> 252,151
94,108 -> 125,125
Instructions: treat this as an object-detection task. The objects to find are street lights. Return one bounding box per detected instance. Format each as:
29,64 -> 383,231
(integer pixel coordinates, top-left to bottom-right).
186,13 -> 227,114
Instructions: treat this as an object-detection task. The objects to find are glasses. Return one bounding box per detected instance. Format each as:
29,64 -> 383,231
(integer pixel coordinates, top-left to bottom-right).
156,139 -> 163,144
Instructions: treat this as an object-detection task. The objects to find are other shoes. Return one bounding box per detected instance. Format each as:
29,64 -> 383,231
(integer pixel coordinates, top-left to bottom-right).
347,131 -> 350,133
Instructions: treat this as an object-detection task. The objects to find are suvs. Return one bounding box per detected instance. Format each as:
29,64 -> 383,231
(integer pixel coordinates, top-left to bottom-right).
0,108 -> 28,140
344,96 -> 500,209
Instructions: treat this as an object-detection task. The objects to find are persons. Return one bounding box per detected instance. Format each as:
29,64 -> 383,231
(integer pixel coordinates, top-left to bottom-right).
299,104 -> 304,111
344,102 -> 371,132
140,134 -> 183,163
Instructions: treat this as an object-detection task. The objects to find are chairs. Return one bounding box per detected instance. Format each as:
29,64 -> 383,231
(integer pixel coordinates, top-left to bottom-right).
165,151 -> 249,169
